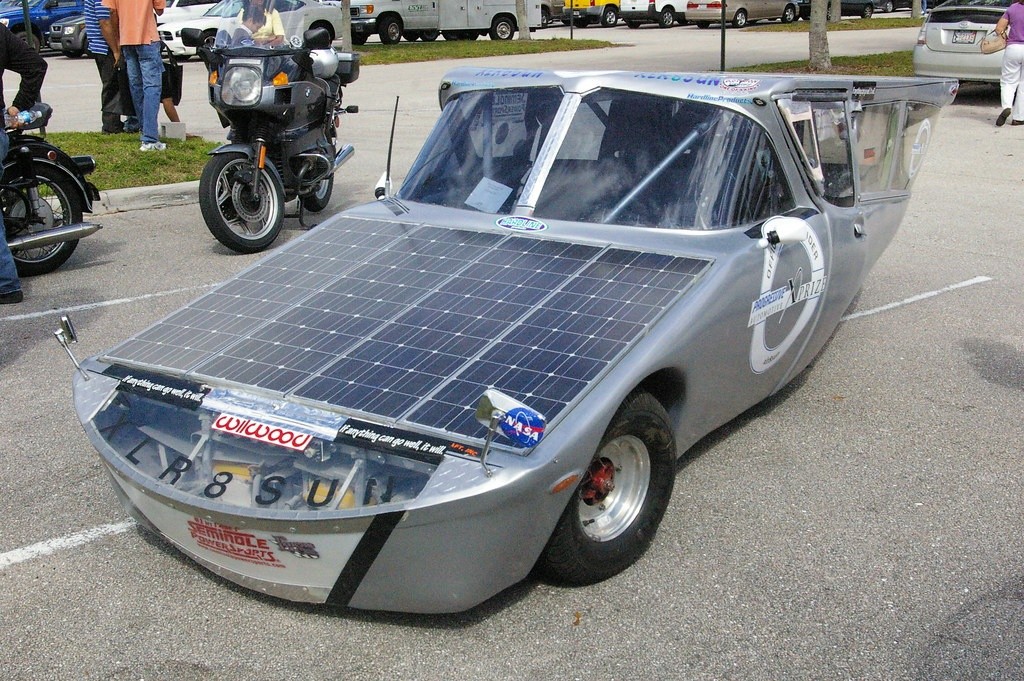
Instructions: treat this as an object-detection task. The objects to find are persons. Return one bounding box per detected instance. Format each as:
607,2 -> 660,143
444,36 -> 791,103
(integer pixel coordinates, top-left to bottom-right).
226,0 -> 292,144
0,0 -> 181,305
995,0 -> 1024,126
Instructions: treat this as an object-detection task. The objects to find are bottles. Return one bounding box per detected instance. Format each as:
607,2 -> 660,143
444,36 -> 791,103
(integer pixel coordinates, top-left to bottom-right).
4,110 -> 42,127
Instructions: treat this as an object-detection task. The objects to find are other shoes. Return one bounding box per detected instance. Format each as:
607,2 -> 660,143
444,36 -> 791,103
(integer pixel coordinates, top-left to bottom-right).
1011,120 -> 1024,125
996,108 -> 1012,126
0,289 -> 24,304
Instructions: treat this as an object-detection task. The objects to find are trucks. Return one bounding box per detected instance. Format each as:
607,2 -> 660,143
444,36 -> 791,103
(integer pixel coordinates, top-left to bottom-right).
329,0 -> 541,47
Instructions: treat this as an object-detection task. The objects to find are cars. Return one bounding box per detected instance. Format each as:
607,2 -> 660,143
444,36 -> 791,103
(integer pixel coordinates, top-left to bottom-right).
0,0 -> 343,61
540,0 -> 943,28
911,1 -> 1018,84
52,64 -> 961,615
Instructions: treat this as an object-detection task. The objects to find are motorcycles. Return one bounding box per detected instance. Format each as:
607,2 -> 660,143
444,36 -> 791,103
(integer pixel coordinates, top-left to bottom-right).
180,28 -> 360,254
1,102 -> 105,276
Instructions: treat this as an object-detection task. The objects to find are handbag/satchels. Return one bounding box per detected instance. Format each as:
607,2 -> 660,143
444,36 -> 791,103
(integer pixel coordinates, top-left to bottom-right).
160,40 -> 183,106
981,29 -> 1007,54
100,55 -> 137,116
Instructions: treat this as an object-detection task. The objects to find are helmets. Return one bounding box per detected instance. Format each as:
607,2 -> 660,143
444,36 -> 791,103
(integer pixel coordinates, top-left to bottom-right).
309,43 -> 338,78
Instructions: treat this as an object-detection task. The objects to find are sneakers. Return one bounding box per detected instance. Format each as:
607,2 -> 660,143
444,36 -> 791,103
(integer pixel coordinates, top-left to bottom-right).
140,141 -> 166,152
